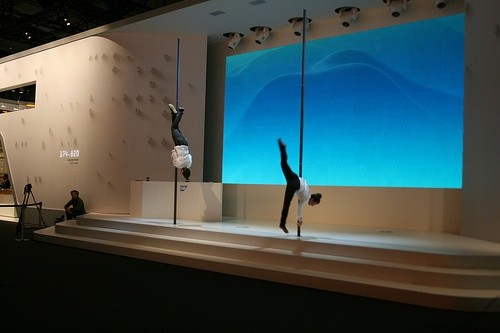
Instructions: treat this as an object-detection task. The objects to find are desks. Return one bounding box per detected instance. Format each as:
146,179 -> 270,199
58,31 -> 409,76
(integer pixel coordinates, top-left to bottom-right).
130,179 -> 223,222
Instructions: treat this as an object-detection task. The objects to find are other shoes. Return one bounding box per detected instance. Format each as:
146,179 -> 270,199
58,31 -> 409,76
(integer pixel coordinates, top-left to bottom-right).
178,106 -> 184,111
168,103 -> 178,116
279,139 -> 285,149
280,223 -> 288,233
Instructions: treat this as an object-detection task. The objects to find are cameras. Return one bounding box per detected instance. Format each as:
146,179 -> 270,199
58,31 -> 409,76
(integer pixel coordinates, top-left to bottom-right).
24,184 -> 32,192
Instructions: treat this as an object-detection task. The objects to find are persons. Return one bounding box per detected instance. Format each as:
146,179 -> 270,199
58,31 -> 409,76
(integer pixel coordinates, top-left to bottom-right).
168,104 -> 192,180
56,190 -> 86,220
0,174 -> 11,189
278,138 -> 322,237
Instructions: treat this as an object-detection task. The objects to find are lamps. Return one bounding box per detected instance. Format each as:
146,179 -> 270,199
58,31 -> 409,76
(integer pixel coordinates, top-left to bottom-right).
386,0 -> 408,18
436,0 -> 448,11
228,33 -> 242,50
339,7 -> 358,29
293,18 -> 311,36
254,27 -> 270,44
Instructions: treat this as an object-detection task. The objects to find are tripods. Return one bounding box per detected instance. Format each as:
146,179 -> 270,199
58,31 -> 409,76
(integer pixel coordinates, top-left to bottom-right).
14,190 -> 48,232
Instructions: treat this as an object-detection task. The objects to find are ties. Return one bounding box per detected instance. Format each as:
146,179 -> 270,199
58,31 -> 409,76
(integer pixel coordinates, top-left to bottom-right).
4,181 -> 5,185
